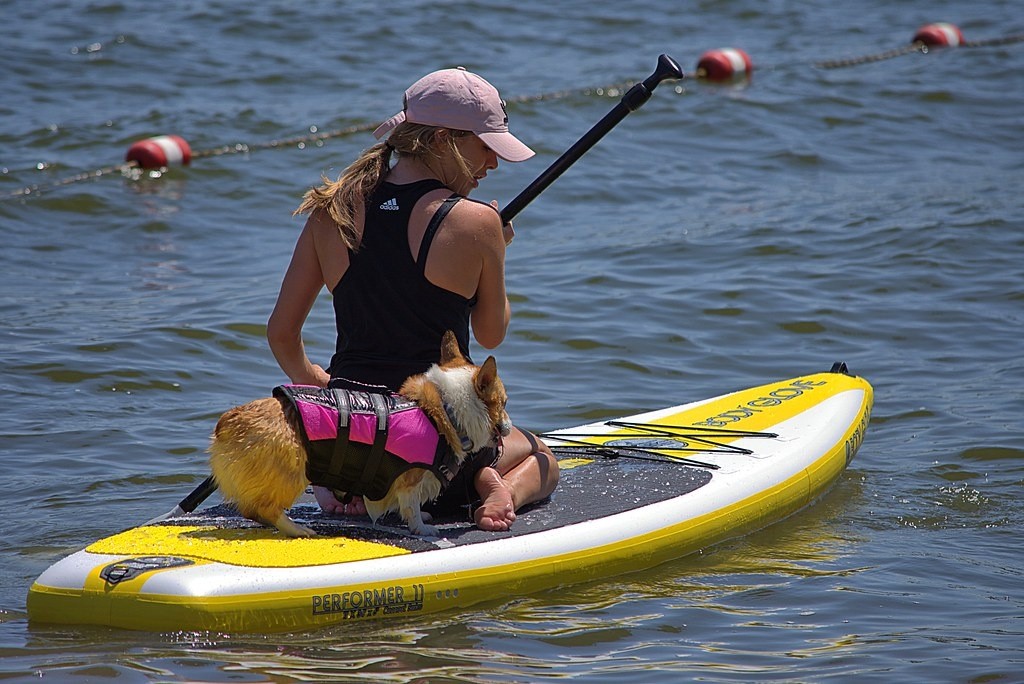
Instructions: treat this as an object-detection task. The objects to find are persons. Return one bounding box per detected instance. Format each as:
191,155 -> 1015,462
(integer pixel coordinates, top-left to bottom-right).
266,66 -> 561,531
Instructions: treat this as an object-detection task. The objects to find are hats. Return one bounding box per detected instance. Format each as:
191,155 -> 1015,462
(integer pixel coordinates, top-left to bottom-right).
371,66 -> 536,163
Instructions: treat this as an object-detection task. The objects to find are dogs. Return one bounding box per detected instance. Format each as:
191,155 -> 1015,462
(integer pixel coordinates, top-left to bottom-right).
204,326 -> 509,539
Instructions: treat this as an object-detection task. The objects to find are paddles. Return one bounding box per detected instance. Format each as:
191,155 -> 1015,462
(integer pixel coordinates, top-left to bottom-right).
133,50 -> 687,524
